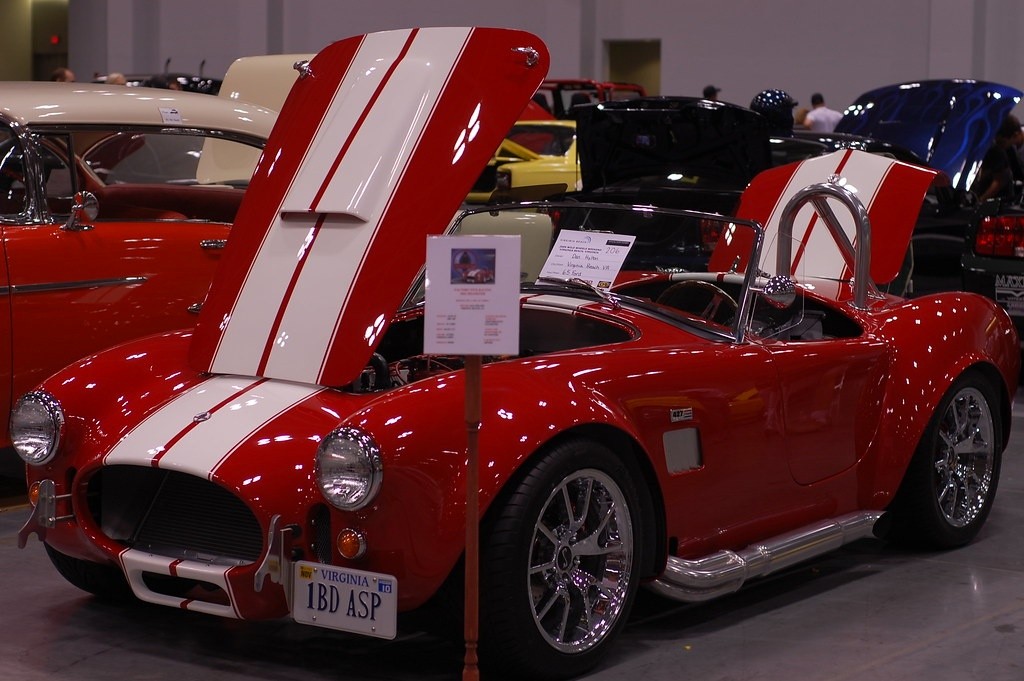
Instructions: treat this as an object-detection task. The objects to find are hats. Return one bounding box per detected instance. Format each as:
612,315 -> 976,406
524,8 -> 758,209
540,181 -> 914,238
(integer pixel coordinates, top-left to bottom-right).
703,85 -> 722,100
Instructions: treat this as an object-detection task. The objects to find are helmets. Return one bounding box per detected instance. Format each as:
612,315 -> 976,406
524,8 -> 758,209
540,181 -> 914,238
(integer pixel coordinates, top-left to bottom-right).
751,88 -> 799,137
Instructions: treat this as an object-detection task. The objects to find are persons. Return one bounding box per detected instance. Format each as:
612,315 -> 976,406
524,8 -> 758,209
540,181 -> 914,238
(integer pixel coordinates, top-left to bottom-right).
751,88 -> 797,138
105,72 -> 126,85
804,93 -> 844,133
795,109 -> 809,125
703,85 -> 721,100
151,76 -> 182,90
51,68 -> 76,83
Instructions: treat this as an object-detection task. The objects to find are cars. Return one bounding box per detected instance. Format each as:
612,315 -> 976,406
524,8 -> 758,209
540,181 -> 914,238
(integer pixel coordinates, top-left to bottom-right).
0,53 -> 555,458
465,75 -> 1024,330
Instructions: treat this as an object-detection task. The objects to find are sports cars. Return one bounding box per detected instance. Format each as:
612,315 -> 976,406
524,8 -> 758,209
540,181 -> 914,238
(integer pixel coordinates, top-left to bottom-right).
6,26 -> 1024,681
